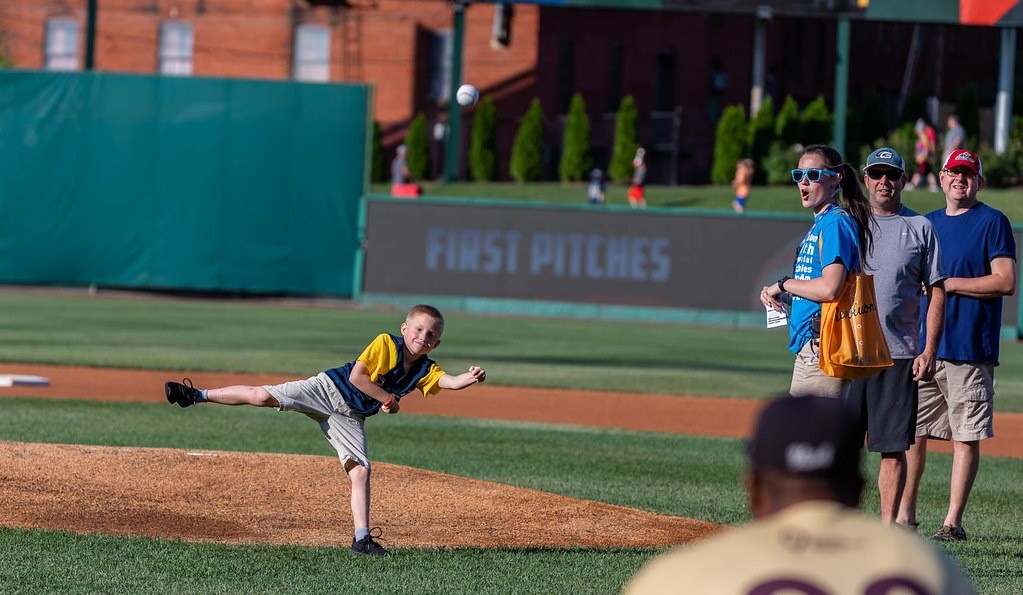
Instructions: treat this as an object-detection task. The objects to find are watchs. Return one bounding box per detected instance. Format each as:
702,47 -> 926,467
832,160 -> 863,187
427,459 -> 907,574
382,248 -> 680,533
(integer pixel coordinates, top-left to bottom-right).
777,275 -> 790,293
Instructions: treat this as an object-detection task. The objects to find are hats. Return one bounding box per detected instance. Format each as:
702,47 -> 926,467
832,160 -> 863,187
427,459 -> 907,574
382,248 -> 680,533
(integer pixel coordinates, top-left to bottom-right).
862,148 -> 906,176
942,149 -> 982,176
747,397 -> 864,477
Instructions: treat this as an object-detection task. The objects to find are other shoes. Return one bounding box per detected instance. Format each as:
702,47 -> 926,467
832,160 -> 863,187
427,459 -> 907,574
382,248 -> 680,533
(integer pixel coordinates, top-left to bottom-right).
931,526 -> 966,542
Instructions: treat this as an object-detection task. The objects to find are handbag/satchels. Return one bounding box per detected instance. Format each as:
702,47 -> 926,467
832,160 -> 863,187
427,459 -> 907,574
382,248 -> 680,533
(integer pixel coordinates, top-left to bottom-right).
818,210 -> 894,377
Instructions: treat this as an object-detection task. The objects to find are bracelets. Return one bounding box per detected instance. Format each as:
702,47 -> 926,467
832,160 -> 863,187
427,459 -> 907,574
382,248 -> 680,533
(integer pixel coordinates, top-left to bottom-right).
382,394 -> 397,409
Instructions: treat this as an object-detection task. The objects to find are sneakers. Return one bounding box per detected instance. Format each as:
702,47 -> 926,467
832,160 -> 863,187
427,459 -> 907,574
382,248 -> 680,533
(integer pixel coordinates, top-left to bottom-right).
350,527 -> 391,557
165,378 -> 203,408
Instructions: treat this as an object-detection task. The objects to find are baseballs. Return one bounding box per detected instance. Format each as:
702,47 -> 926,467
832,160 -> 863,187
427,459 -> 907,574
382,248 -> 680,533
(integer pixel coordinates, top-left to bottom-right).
456,83 -> 479,106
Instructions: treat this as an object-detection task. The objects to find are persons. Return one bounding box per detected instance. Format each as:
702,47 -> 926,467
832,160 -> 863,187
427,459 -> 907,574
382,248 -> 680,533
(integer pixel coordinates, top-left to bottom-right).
165,303 -> 486,558
760,115 -> 1017,544
626,149 -> 651,210
616,394 -> 984,595
388,143 -> 415,199
730,157 -> 753,215
586,167 -> 607,205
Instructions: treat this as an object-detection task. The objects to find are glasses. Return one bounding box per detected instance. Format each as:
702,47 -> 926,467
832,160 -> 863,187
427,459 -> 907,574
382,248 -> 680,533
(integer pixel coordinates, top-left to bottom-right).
790,167 -> 837,183
944,168 -> 975,180
864,167 -> 902,181
